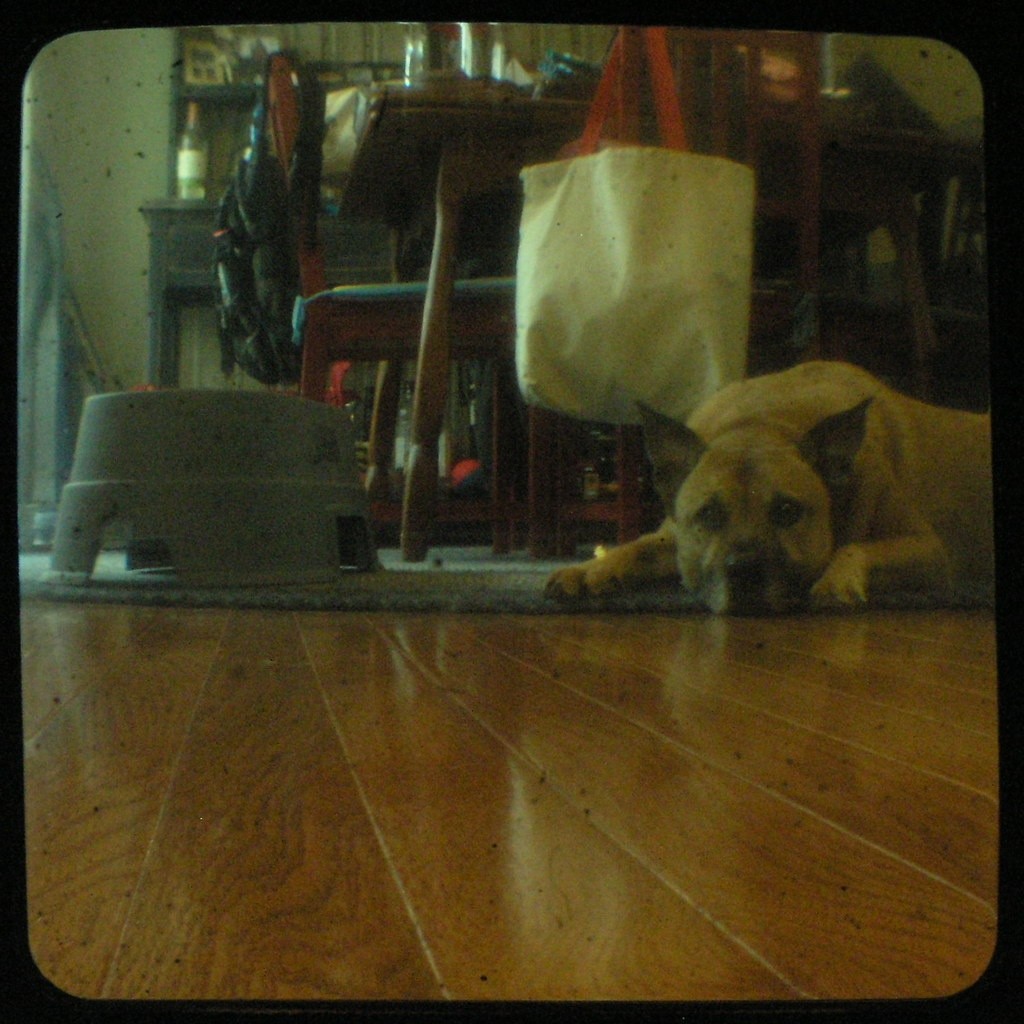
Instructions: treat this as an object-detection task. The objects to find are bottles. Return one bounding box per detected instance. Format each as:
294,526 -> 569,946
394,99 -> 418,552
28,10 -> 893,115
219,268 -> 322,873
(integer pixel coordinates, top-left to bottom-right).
173,100 -> 210,201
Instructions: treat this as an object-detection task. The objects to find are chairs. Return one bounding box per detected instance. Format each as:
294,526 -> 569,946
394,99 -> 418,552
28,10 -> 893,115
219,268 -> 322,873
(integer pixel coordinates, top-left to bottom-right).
267,51 -> 547,557
527,25 -> 821,557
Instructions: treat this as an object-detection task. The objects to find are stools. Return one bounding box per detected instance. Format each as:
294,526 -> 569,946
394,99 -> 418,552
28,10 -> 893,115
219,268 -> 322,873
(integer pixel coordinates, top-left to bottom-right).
50,389 -> 374,587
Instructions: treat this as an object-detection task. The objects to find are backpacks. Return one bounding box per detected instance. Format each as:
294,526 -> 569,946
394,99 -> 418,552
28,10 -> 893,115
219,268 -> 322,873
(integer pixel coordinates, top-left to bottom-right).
209,48 -> 327,386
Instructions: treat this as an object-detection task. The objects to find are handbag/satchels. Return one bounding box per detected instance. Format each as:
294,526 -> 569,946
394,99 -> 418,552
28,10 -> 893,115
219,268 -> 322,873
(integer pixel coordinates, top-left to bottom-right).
516,24 -> 756,427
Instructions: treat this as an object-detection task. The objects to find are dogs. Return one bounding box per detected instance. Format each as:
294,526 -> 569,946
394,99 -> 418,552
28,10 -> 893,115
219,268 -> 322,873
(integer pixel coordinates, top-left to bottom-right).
541,363 -> 993,618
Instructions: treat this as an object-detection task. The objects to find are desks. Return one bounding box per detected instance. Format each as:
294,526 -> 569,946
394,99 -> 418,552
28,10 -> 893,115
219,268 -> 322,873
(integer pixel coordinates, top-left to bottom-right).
333,81 -> 941,560
138,201 -> 224,388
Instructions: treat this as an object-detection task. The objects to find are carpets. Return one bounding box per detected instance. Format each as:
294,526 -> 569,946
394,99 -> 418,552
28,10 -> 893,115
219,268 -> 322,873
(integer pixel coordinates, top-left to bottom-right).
18,534 -> 994,615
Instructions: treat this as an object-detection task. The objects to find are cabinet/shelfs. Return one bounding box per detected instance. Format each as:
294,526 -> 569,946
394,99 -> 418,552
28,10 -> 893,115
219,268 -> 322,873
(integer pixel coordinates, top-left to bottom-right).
164,26 -> 283,202
